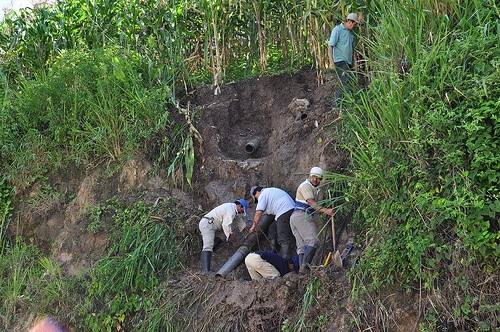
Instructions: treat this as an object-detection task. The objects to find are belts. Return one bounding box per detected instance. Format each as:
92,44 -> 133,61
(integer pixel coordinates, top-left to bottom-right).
203,217 -> 209,219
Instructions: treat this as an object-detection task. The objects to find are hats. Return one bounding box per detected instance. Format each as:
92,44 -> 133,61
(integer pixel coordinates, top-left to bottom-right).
236,198 -> 248,217
310,167 -> 324,178
250,186 -> 259,204
291,255 -> 299,271
346,13 -> 361,23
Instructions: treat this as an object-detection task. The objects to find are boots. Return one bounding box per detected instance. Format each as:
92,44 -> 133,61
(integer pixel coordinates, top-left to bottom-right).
299,245 -> 317,275
282,244 -> 291,258
200,250 -> 212,273
269,238 -> 277,252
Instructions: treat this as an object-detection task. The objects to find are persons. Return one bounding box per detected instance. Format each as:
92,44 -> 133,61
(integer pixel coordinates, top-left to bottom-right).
199,198 -> 253,274
250,186 -> 296,259
327,13 -> 360,108
245,251 -> 298,281
290,167 -> 334,274
